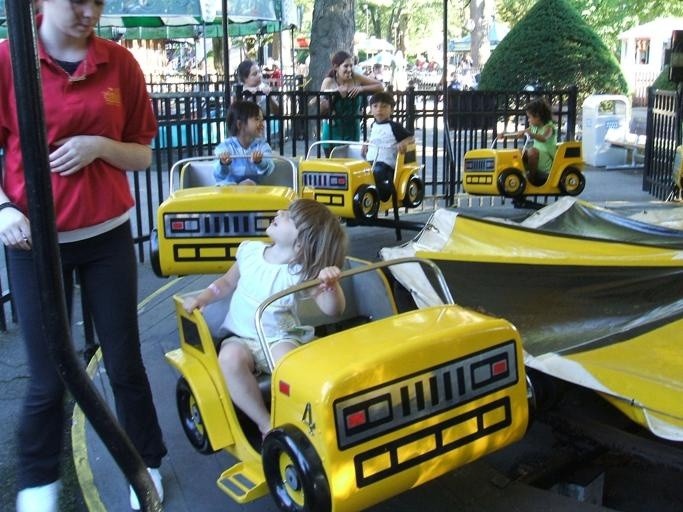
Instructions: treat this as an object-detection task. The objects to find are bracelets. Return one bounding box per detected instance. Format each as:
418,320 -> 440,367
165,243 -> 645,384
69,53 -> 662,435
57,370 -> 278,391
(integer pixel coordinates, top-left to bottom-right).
0,202 -> 22,212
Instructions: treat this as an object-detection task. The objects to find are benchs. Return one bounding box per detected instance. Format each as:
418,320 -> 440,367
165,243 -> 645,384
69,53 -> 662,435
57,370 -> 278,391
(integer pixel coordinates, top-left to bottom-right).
605,132 -> 678,170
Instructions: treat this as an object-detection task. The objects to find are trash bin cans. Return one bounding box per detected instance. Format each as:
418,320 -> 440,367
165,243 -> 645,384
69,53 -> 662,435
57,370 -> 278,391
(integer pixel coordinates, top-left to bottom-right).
582,94 -> 631,168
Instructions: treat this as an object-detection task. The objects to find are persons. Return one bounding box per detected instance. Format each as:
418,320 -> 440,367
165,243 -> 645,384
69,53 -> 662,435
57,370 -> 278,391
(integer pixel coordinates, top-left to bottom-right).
180,197 -> 349,443
364,91 -> 413,184
228,57 -> 281,117
261,62 -> 481,91
211,100 -> 275,187
496,97 -> 558,184
317,51 -> 384,158
0,1 -> 169,511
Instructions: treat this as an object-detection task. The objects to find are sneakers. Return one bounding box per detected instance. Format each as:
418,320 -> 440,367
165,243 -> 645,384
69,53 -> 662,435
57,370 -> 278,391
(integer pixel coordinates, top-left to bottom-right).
128,467 -> 164,510
